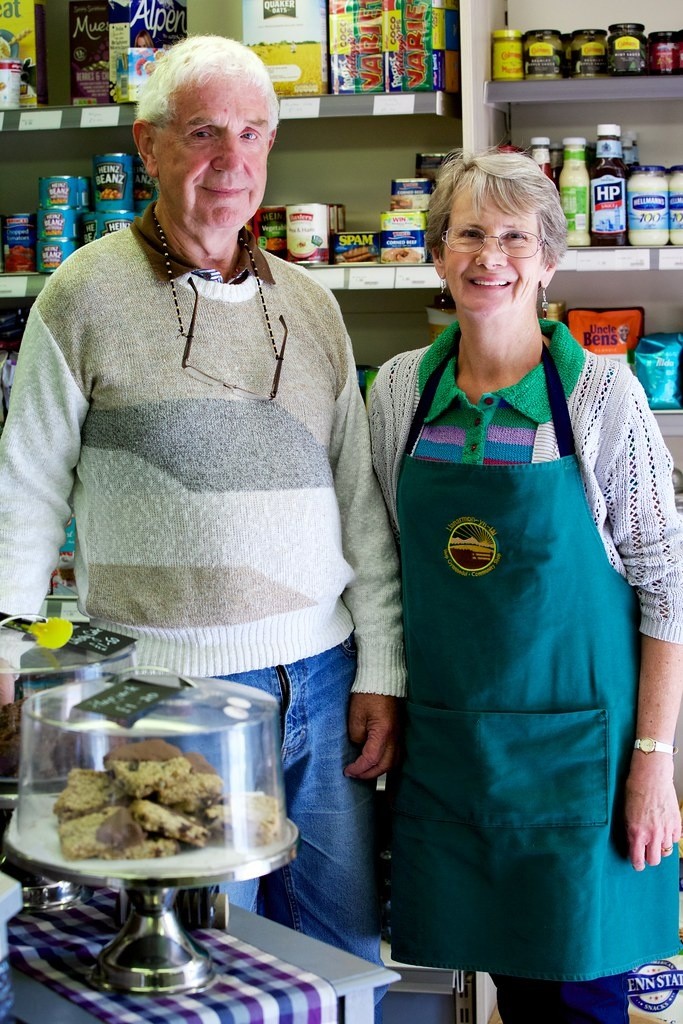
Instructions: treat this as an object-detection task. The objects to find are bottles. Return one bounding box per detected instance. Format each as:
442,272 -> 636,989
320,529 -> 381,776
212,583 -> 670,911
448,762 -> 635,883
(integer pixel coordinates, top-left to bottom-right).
495,124 -> 683,246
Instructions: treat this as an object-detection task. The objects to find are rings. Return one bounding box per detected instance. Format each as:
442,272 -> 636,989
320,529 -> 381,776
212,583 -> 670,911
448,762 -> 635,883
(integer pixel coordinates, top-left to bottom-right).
660,842 -> 674,853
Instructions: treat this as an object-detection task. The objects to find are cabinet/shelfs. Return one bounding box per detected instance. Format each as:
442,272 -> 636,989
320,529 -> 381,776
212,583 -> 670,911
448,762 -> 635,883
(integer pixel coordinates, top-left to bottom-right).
0,0 -> 683,625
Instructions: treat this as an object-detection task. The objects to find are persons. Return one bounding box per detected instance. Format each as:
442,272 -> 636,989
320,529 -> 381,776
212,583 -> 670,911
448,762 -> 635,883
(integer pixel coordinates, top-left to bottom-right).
366,147 -> 679,1024
1,32 -> 402,1015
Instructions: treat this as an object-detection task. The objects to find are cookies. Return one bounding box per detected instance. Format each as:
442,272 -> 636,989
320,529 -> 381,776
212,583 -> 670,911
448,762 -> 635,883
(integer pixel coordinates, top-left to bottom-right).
51,739 -> 278,863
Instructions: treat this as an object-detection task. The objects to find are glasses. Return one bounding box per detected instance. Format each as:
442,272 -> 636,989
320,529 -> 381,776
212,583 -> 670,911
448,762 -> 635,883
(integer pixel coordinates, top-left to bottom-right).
174,275 -> 290,401
436,224 -> 545,258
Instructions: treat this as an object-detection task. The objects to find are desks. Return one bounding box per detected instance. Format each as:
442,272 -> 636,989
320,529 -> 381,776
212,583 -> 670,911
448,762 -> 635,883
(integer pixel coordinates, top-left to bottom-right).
0,879 -> 404,1023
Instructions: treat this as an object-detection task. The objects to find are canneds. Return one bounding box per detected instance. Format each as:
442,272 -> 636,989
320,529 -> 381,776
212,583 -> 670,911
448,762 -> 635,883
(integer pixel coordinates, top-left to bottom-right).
0,149 -> 448,271
490,20 -> 682,82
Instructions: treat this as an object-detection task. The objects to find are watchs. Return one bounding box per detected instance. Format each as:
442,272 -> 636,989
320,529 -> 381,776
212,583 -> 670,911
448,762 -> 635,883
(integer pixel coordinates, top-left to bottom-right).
634,734 -> 676,756
